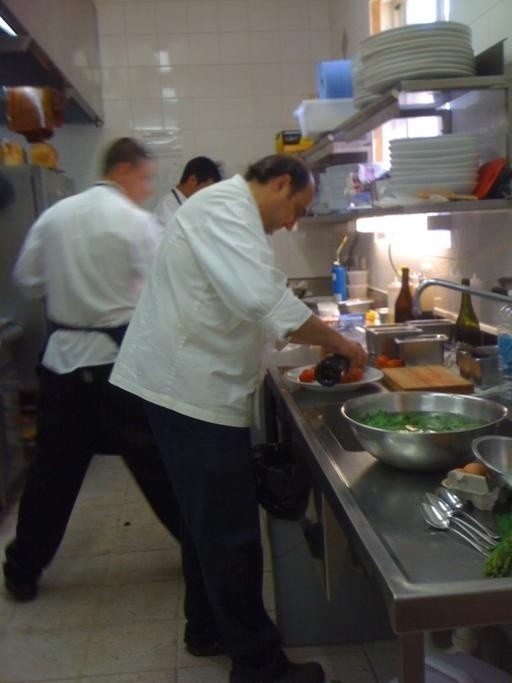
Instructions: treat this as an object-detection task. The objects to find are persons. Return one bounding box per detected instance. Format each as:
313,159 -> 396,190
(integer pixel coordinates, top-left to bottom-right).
109,153 -> 372,683
4,136 -> 167,602
153,156 -> 227,232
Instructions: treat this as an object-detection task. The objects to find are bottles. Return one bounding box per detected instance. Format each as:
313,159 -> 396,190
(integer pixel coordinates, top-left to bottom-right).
456,278 -> 481,347
314,352 -> 353,386
394,267 -> 414,325
497,290 -> 511,379
331,263 -> 347,301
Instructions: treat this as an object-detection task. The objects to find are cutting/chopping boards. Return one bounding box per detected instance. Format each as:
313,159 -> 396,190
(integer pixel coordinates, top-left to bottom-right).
381,363 -> 474,395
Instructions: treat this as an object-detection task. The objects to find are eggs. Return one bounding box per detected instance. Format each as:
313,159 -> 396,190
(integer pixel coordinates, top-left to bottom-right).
464,463 -> 486,475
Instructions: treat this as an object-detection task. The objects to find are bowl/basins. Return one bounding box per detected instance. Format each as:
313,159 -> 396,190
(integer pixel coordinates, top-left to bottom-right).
342,392 -> 512,492
346,270 -> 370,298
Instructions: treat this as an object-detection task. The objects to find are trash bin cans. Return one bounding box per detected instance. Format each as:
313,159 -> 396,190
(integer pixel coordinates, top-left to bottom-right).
253,440 -> 383,645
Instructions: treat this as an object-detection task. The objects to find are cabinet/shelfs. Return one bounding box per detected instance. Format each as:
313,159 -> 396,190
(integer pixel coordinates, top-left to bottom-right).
292,76 -> 512,228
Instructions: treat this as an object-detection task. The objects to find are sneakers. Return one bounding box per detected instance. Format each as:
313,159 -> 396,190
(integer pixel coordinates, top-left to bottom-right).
274,662 -> 324,681
186,643 -> 225,657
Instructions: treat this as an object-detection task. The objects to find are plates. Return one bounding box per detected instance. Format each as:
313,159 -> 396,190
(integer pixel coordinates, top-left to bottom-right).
286,361 -> 383,388
387,135 -> 481,195
349,22 -> 478,110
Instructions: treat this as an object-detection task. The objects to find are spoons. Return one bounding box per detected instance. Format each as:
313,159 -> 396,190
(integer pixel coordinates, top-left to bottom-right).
422,487 -> 501,556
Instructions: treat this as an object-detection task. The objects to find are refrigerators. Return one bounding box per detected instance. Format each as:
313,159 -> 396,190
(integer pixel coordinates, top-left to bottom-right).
0,162 -> 78,358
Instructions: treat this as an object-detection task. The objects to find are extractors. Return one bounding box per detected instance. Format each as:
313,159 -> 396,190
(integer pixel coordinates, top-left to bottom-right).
1,1 -> 105,128
